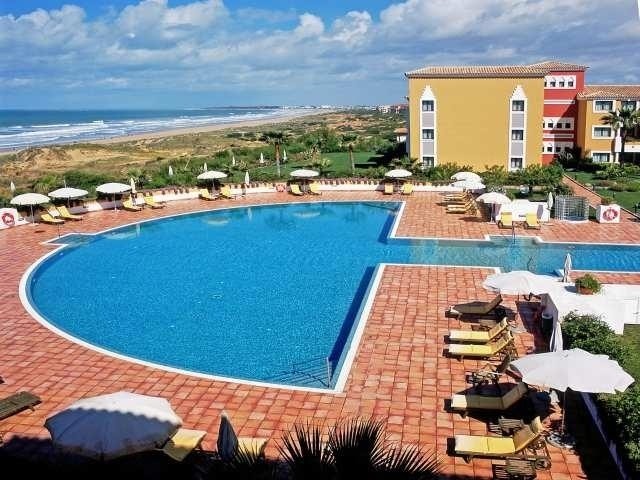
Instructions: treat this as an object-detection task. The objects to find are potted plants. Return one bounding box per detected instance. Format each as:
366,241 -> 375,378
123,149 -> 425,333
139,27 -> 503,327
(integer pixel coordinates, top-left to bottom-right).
575,274 -> 601,296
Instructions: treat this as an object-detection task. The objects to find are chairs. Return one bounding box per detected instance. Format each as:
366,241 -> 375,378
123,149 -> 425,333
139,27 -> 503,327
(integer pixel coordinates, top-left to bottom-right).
447,293 -> 552,464
384,183 -> 413,195
146,428 -> 270,465
446,190 -> 475,213
200,186 -> 237,200
290,184 -> 322,195
497,211 -> 541,230
37,205 -> 84,225
122,195 -> 165,212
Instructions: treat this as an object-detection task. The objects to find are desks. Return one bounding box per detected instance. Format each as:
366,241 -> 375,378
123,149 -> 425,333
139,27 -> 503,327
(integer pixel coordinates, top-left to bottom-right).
505,458 -> 537,480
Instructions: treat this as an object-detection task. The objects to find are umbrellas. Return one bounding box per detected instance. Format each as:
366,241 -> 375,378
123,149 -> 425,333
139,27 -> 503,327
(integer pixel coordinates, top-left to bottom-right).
95,181 -> 132,208
290,169 -> 319,191
45,390 -> 182,461
546,191 -> 554,223
385,169 -> 412,192
10,192 -> 50,223
48,186 -> 89,208
131,178 -> 137,206
196,169 -> 227,193
475,191 -> 511,222
450,180 -> 486,196
509,348 -> 634,439
548,319 -> 563,353
561,253 -> 572,283
450,171 -> 481,182
483,267 -> 558,327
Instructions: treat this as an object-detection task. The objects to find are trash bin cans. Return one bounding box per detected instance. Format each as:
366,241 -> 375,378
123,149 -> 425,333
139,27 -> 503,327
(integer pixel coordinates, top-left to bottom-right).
541,312 -> 553,336
